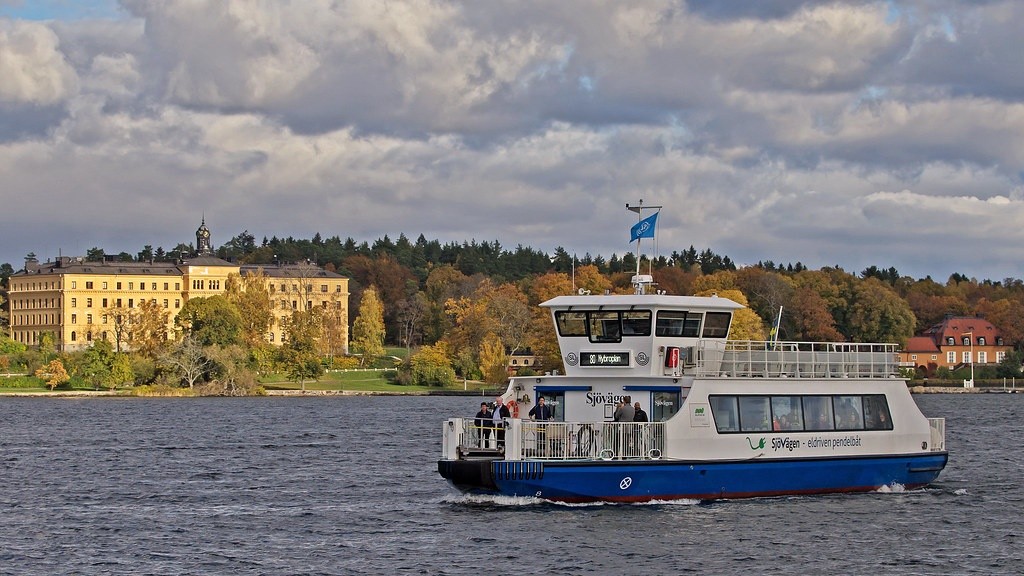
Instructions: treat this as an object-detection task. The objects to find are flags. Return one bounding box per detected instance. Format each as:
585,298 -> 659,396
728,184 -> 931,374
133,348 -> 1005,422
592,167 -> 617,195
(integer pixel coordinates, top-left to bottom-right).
629,212 -> 658,243
769,312 -> 779,351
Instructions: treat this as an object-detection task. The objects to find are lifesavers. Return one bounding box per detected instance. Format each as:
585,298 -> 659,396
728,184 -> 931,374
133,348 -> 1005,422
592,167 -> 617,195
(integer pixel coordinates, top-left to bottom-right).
507,401 -> 519,418
576,424 -> 593,458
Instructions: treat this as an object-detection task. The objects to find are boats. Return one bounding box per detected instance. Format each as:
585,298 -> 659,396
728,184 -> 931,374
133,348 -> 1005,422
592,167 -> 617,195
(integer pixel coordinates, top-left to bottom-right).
438,198 -> 948,504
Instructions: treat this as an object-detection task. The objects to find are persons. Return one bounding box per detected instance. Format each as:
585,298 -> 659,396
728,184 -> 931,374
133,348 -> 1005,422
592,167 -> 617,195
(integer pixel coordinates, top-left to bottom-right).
614,320 -> 634,341
839,398 -> 861,428
612,396 -> 648,459
490,398 -> 512,450
813,413 -> 830,429
474,402 -> 493,450
528,397 -> 555,450
773,414 -> 798,430
873,414 -> 889,429
